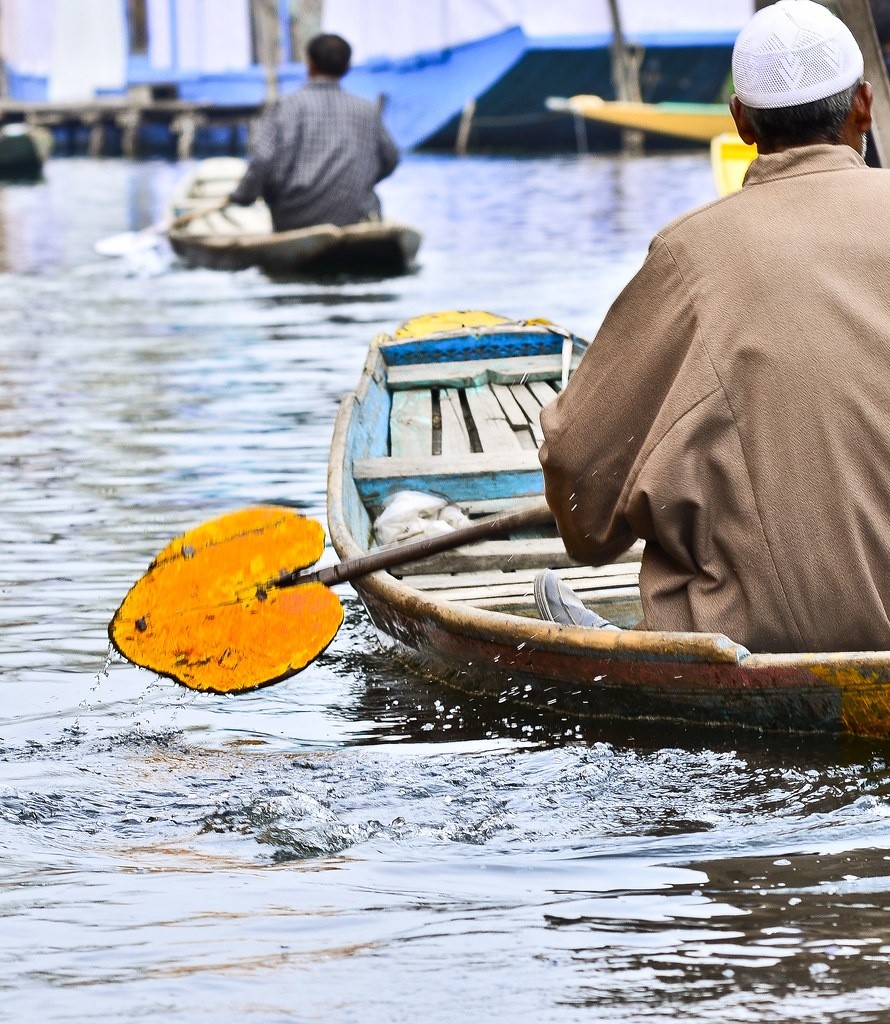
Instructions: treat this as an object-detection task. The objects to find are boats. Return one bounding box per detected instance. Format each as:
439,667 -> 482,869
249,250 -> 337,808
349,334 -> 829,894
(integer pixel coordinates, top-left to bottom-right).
160,156 -> 423,285
321,302 -> 890,746
0,118 -> 57,182
537,89 -> 739,149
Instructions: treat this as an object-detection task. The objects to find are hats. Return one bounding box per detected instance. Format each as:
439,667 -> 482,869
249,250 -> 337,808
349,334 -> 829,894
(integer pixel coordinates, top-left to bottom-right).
731,0 -> 864,107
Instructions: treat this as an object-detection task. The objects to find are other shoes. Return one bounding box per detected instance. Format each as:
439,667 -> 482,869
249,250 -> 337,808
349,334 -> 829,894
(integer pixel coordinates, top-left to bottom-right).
533,568 -> 610,627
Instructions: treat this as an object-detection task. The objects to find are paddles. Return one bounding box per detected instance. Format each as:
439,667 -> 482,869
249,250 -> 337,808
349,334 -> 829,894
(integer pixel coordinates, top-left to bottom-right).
92,199 -> 230,259
110,477 -> 562,694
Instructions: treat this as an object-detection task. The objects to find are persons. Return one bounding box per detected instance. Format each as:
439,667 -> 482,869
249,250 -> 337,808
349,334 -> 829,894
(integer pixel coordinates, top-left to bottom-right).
537,0 -> 890,654
227,33 -> 400,234
